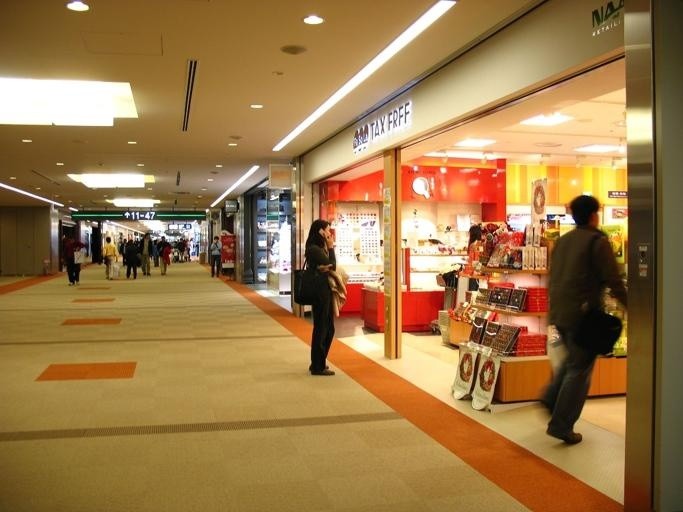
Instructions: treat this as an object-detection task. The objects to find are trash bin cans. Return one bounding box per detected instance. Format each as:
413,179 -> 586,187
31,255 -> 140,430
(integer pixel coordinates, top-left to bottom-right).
43,256 -> 52,275
199,251 -> 206,264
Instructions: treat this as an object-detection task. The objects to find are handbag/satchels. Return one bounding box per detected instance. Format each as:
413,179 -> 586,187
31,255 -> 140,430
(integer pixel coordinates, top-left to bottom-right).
293,250 -> 316,305
111,261 -> 121,279
73,249 -> 83,264
566,308 -> 621,354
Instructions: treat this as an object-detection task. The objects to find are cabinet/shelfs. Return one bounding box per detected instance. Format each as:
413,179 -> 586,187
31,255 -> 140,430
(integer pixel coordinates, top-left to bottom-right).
360,247 -> 469,333
439,247 -> 626,402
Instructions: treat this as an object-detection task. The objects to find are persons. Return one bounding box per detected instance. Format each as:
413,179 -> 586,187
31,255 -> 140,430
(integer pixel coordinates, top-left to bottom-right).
536,195 -> 627,443
100,232 -> 198,280
459,225 -> 483,276
209,235 -> 222,277
302,219 -> 336,376
62,233 -> 84,286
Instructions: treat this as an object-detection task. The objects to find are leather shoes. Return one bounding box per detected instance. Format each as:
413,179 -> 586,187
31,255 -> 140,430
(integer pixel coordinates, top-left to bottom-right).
546,429 -> 581,444
311,370 -> 335,375
310,363 -> 329,370
